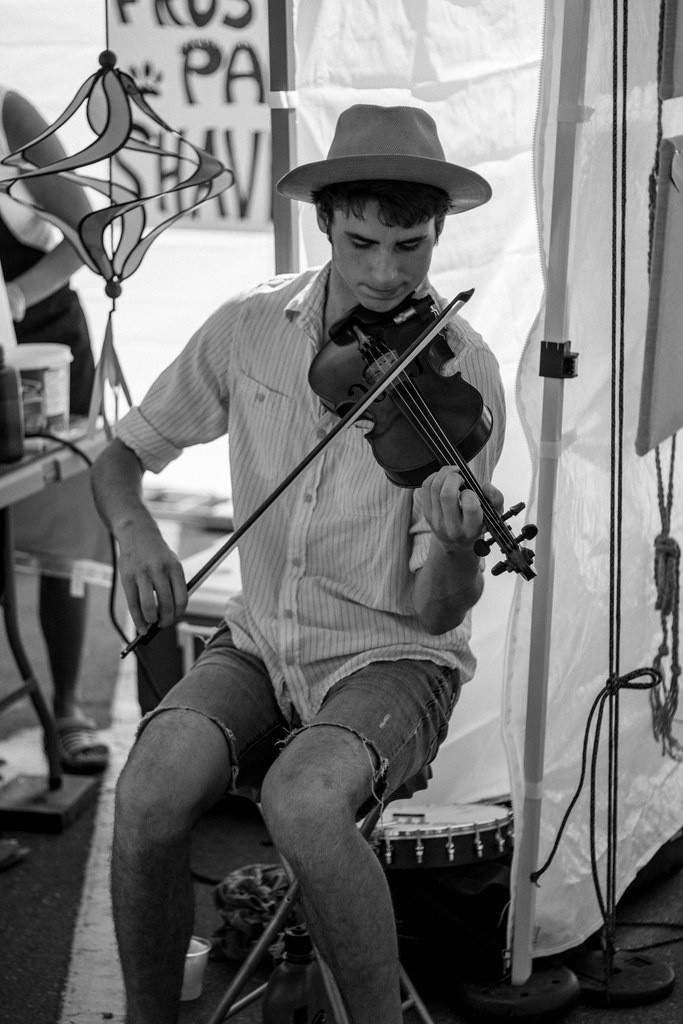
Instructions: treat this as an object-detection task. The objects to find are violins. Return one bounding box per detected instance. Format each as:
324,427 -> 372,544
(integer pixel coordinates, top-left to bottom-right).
307,291 -> 540,582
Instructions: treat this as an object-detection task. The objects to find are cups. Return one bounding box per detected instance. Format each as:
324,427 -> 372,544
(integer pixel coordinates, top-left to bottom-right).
178,936 -> 214,1002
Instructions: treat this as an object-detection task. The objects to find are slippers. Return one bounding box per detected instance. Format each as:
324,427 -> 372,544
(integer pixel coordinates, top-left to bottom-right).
49,715 -> 111,775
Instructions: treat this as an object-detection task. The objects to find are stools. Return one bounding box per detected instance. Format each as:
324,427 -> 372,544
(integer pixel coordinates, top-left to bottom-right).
206,755 -> 432,1023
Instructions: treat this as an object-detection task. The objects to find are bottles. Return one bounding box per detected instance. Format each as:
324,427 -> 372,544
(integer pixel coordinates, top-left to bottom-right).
0,346 -> 25,465
259,923 -> 333,1024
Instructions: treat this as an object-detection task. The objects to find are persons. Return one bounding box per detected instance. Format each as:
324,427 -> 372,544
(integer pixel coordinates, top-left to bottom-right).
85,102 -> 509,1022
0,83 -> 115,774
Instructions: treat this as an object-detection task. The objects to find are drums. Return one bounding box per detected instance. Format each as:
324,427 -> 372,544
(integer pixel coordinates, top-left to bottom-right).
355,803 -> 516,998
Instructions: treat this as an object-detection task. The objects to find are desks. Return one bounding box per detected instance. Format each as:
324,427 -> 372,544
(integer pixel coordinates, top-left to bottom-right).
0,418 -> 130,795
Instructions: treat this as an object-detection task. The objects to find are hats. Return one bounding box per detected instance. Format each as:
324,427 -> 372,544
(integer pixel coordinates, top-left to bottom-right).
276,102 -> 493,218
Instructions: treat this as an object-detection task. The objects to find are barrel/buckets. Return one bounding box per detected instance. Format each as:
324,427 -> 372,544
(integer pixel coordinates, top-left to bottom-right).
17,343 -> 76,444
